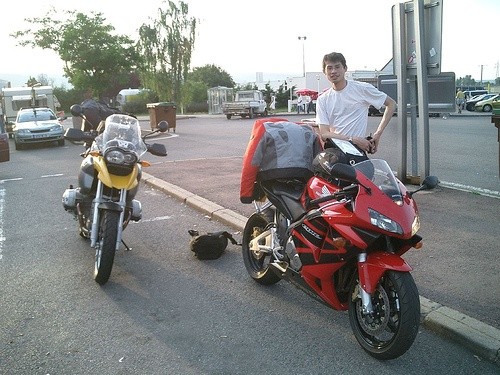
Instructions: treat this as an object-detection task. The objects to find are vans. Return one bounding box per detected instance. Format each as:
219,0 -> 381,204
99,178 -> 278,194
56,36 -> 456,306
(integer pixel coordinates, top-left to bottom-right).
462,89 -> 489,101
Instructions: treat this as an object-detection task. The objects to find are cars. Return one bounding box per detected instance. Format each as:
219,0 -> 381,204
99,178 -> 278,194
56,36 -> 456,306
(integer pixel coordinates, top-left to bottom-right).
9,107 -> 65,151
465,94 -> 496,111
474,94 -> 500,112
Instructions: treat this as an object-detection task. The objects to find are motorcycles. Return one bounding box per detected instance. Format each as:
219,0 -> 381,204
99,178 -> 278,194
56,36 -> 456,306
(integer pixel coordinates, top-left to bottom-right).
62,104 -> 169,285
241,137 -> 439,360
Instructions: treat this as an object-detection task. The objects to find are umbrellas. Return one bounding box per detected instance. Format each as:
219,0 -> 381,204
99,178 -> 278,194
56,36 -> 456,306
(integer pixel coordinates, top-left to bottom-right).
295,89 -> 315,96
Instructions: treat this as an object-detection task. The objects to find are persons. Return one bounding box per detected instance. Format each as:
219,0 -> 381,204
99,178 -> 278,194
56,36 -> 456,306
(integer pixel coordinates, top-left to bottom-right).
297,93 -> 316,114
456,88 -> 465,113
316,52 -> 396,181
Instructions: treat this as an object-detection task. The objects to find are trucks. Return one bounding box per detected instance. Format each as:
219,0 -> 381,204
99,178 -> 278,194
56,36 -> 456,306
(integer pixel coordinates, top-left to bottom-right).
221,90 -> 268,120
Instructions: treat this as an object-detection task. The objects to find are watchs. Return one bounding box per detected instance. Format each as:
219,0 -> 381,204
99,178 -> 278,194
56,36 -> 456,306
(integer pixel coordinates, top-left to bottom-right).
348,136 -> 353,144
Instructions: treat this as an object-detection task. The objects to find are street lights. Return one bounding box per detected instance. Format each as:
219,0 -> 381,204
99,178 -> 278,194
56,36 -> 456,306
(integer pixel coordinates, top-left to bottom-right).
297,36 -> 306,78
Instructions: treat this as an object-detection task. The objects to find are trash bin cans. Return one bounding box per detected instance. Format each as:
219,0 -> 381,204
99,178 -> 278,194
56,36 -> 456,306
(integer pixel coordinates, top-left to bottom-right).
146,102 -> 177,128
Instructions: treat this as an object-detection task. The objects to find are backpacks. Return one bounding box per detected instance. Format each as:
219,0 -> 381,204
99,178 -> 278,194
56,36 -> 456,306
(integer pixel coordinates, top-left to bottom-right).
188,228 -> 242,260
